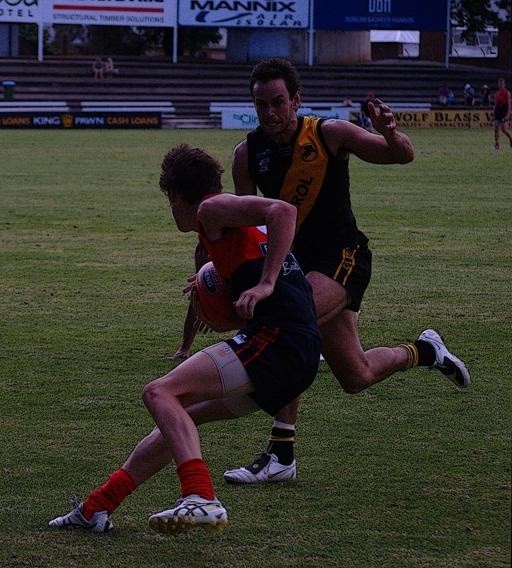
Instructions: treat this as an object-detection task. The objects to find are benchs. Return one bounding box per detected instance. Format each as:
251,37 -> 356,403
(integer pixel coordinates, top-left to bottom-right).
0,56 -> 512,129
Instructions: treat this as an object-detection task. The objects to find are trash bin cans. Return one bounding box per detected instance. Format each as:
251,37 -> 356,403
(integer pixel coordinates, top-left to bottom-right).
2,81 -> 16,98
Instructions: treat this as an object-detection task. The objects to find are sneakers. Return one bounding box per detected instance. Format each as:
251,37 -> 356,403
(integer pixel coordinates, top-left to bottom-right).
48,504 -> 117,534
416,328 -> 472,390
221,453 -> 298,486
147,497 -> 228,535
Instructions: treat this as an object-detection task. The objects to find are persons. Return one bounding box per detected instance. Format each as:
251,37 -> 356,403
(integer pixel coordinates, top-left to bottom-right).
182,57 -> 472,485
47,142 -> 327,535
92,55 -> 104,79
342,77 -> 512,150
102,56 -> 113,79
171,243 -> 212,359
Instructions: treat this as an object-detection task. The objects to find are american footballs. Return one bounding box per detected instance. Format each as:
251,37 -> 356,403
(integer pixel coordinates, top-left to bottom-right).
196,260 -> 239,322
